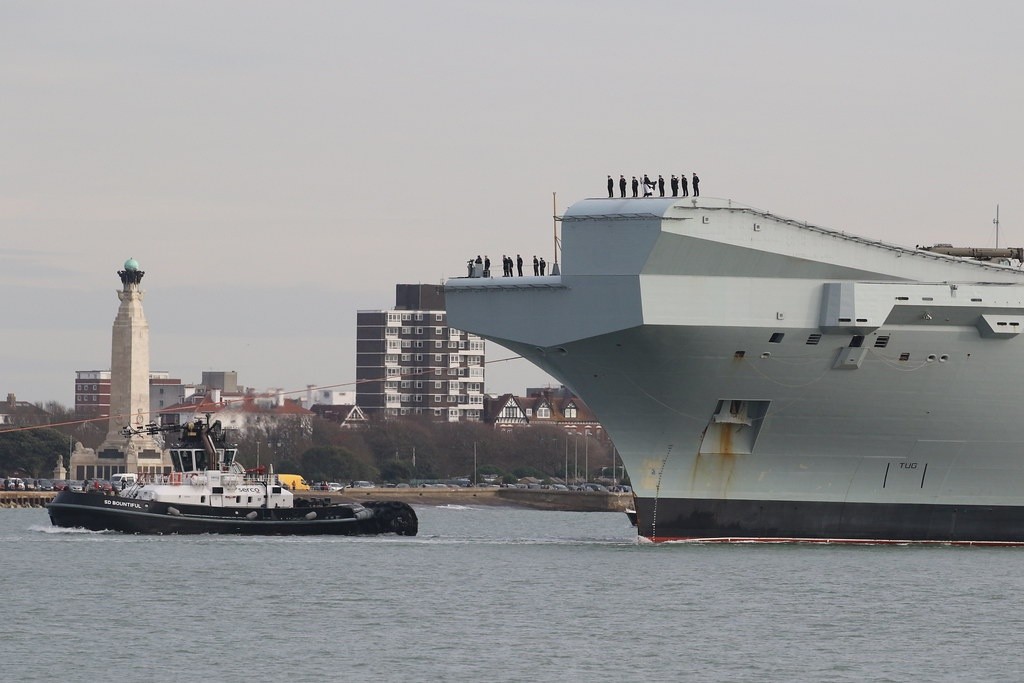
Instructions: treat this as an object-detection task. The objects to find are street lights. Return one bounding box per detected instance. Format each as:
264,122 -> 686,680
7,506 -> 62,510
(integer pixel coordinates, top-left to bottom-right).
564,431 -> 573,486
574,431 -> 582,484
584,432 -> 592,482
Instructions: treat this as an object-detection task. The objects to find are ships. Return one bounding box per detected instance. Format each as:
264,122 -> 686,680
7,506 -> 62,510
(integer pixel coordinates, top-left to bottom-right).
438,191 -> 1023,537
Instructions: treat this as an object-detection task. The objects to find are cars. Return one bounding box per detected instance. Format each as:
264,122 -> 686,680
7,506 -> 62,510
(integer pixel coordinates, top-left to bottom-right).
312,479 -> 632,492
0,472 -> 139,491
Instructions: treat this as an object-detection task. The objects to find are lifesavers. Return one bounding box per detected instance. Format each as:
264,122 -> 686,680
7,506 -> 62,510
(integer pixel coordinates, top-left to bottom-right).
169,472 -> 183,484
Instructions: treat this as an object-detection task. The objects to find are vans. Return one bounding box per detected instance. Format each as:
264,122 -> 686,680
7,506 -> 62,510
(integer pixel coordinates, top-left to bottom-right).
277,474 -> 311,491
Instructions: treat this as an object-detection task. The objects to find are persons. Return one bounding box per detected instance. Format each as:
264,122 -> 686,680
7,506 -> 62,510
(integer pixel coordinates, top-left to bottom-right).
475,254 -> 546,277
608,173 -> 699,197
3,475 -> 627,498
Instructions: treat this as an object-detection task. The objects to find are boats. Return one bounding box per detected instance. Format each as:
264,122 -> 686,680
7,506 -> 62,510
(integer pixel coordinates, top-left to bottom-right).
48,413 -> 420,539
